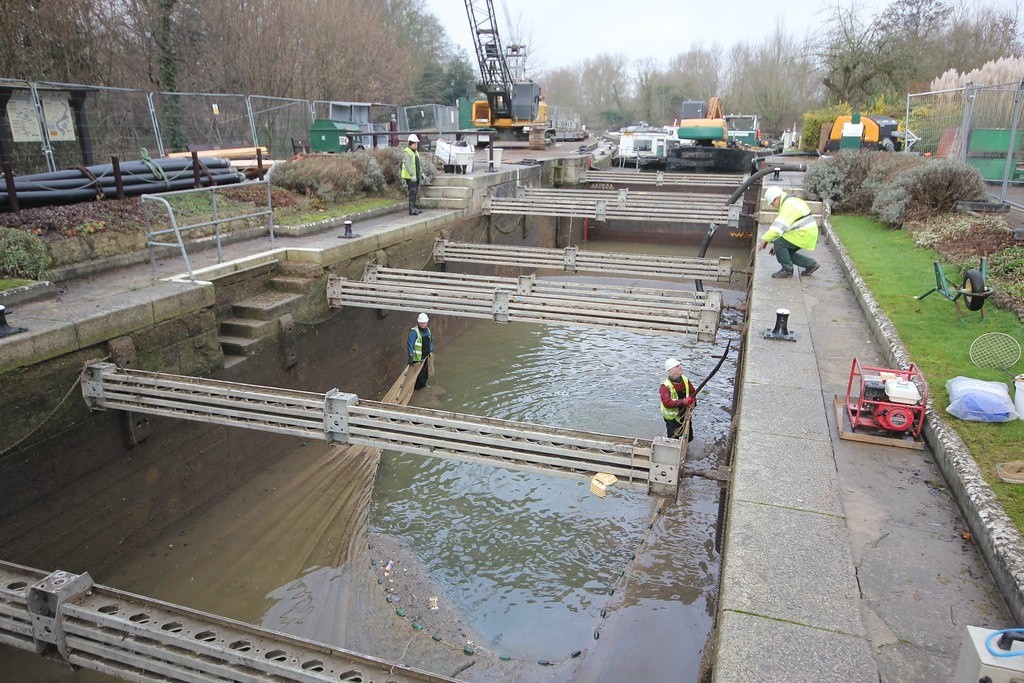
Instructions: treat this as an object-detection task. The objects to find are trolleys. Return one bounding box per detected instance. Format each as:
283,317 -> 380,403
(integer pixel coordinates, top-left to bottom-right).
915,256 -> 995,326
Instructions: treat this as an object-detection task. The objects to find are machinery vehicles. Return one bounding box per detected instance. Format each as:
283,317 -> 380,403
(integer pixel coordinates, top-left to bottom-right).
456,0 -> 557,150
677,96 -> 769,151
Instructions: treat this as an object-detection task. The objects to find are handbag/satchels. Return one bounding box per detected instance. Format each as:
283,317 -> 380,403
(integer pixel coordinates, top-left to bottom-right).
945,376 -> 1019,422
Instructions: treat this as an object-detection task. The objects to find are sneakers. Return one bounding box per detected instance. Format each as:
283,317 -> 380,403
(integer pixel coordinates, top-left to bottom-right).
772,269 -> 793,278
801,262 -> 820,276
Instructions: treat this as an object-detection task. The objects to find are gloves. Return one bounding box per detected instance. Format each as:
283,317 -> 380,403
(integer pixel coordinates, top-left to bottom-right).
408,356 -> 414,366
420,170 -> 425,180
410,173 -> 417,182
682,396 -> 696,406
769,246 -> 775,257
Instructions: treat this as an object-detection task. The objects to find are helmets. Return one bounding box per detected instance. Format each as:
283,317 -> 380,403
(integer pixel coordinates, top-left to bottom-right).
417,312 -> 429,323
764,186 -> 783,206
665,358 -> 682,373
408,134 -> 420,144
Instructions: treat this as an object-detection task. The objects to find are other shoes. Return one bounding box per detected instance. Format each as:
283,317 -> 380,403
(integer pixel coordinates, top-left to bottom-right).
409,208 -> 419,215
414,207 -> 423,213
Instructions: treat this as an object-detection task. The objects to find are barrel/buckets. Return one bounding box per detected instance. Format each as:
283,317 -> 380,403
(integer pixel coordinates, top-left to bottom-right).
1014,380 -> 1024,420
455,152 -> 475,173
483,149 -> 504,168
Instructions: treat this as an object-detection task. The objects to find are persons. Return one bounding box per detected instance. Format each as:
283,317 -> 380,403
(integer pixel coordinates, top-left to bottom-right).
756,186 -> 820,278
660,359 -> 697,443
401,133 -> 422,216
407,313 -> 434,390
